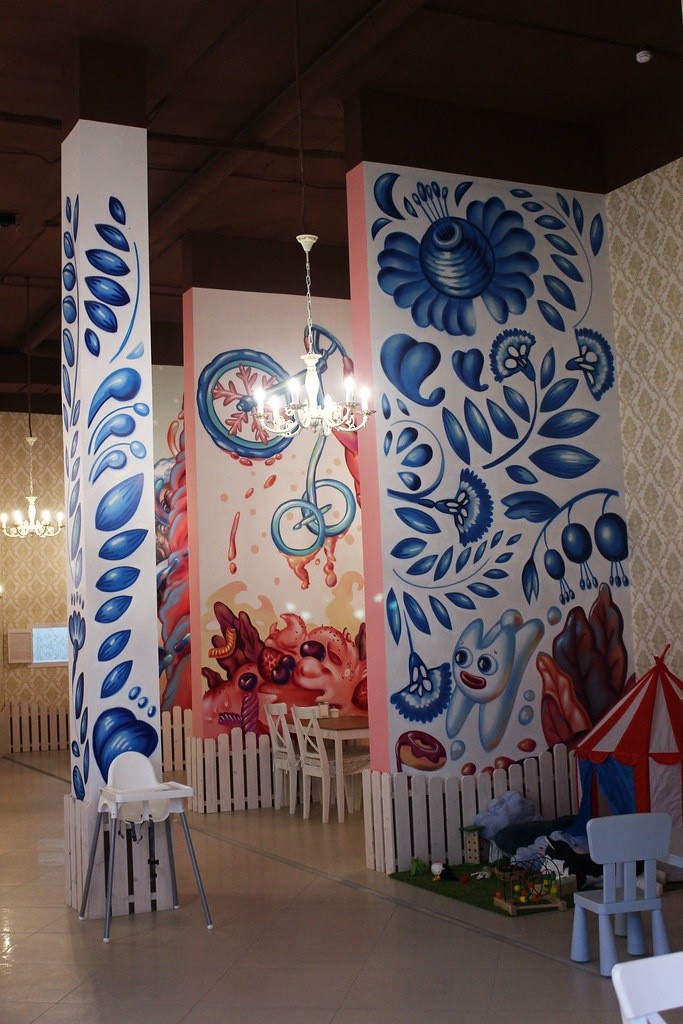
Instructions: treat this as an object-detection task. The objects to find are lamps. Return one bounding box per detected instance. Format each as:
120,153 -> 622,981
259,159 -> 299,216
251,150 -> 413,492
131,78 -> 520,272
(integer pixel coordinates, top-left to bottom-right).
0,437 -> 65,539
252,234 -> 376,437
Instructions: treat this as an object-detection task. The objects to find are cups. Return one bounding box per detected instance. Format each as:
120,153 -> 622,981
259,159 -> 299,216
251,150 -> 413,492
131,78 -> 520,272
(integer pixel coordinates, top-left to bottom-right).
330,707 -> 339,718
319,702 -> 328,717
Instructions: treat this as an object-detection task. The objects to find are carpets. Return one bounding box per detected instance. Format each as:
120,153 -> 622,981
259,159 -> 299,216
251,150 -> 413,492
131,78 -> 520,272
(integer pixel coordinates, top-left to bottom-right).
389,862 -> 589,918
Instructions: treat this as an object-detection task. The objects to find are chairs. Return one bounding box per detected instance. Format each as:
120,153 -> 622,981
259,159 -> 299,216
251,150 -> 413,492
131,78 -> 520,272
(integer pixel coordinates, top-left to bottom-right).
264,702 -> 334,816
290,704 -> 370,825
611,951 -> 683,1024
570,813 -> 671,976
107,751 -> 171,824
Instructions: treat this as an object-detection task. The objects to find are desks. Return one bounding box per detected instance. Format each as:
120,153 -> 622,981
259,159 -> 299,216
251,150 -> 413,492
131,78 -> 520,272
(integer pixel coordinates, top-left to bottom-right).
282,716 -> 369,824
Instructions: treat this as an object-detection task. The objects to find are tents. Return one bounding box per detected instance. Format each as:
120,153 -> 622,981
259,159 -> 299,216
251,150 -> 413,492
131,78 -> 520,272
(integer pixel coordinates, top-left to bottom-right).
549,643 -> 683,883
